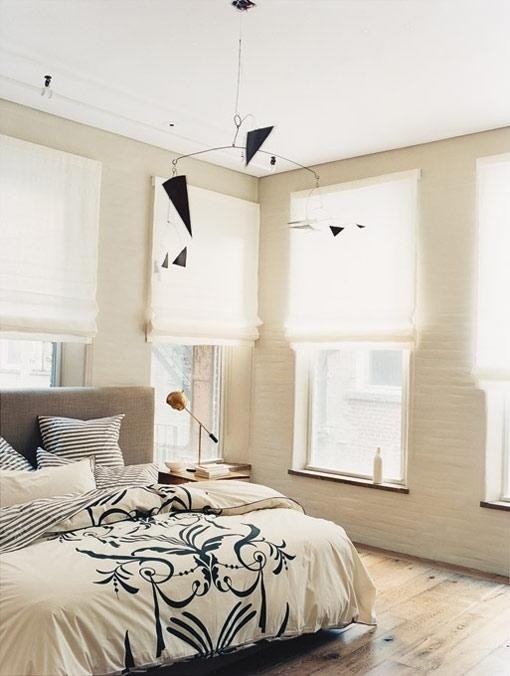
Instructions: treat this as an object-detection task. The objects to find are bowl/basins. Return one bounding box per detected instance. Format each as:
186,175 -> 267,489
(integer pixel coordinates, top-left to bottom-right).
165,462 -> 188,472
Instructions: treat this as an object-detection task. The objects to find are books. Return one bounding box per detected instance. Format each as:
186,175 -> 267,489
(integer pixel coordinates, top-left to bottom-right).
195,464 -> 231,479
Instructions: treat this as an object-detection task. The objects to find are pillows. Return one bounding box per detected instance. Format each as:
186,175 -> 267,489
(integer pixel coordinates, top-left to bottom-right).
0,411 -> 163,508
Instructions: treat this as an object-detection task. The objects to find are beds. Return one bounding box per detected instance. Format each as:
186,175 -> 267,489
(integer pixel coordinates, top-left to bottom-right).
0,383 -> 367,675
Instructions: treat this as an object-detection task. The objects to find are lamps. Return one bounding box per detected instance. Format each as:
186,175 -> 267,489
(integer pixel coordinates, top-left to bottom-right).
166,390 -> 222,472
140,0 -> 365,282
